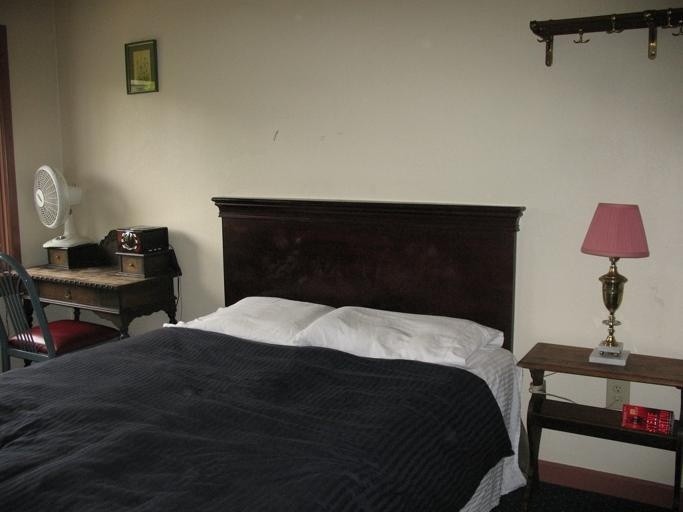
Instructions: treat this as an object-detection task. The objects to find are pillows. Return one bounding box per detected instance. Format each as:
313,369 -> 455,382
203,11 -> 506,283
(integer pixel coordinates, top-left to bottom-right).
163,296 -> 337,346
288,306 -> 505,370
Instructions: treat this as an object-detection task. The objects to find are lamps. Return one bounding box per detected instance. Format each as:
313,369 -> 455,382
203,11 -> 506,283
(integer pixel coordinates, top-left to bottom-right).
580,202 -> 649,368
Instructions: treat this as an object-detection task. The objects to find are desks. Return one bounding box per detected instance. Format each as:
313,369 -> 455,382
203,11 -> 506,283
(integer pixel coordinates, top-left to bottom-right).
0,243 -> 178,372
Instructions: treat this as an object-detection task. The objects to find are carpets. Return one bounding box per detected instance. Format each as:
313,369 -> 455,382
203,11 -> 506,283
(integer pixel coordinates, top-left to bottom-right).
487,478 -> 676,512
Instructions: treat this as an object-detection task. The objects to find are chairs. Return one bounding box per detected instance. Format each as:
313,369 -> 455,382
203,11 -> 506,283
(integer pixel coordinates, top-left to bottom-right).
0,251 -> 123,373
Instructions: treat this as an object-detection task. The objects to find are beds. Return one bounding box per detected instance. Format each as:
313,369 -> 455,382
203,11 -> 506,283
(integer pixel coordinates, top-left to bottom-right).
0,198 -> 525,512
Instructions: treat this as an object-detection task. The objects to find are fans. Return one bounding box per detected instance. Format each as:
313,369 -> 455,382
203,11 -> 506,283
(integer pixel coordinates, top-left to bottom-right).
32,164 -> 97,252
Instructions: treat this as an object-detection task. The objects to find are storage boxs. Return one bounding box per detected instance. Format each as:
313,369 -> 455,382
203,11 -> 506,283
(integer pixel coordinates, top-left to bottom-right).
115,226 -> 168,253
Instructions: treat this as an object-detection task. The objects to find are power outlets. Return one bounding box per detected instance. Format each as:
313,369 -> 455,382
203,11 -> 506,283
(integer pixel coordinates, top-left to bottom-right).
605,378 -> 630,412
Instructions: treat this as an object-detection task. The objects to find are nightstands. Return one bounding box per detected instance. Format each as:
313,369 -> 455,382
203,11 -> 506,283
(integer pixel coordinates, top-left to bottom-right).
515,342 -> 682,511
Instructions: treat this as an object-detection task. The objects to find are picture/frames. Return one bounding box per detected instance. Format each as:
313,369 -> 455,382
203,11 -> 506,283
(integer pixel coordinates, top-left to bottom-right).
125,40 -> 159,95
